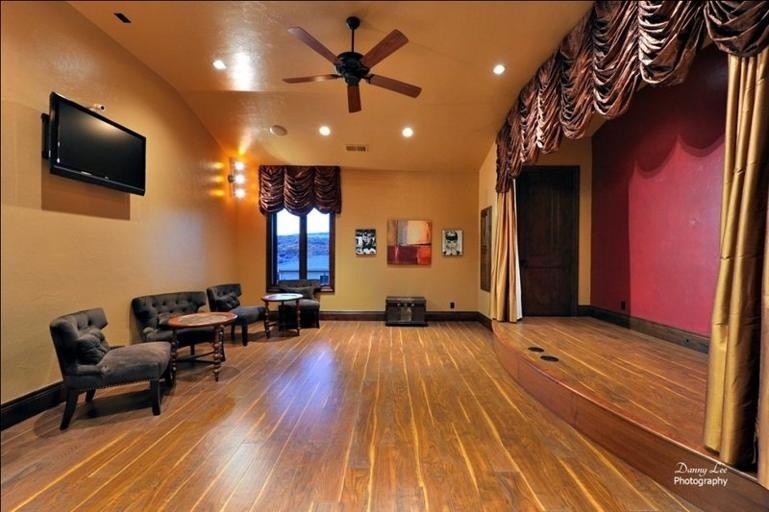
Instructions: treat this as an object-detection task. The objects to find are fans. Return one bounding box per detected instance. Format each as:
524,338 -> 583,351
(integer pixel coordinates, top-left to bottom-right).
282,15 -> 422,113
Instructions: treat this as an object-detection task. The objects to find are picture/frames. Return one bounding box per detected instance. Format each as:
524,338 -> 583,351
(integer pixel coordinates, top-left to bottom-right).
480,205 -> 492,293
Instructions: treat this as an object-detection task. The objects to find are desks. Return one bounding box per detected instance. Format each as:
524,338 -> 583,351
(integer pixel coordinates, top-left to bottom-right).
261,293 -> 304,339
168,311 -> 238,382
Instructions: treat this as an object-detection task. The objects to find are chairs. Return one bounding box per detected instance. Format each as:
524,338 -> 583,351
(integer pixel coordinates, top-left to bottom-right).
206,283 -> 269,346
277,279 -> 321,331
132,291 -> 225,363
49,307 -> 171,431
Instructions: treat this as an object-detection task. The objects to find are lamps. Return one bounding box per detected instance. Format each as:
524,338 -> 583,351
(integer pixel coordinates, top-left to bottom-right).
229,174 -> 245,184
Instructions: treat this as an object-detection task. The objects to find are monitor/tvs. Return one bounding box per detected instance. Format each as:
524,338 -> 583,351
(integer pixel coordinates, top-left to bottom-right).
49,92 -> 146,196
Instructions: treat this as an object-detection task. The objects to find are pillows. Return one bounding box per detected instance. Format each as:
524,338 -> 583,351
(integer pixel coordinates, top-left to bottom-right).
76,327 -> 110,365
157,312 -> 182,327
216,292 -> 240,311
284,286 -> 314,300
